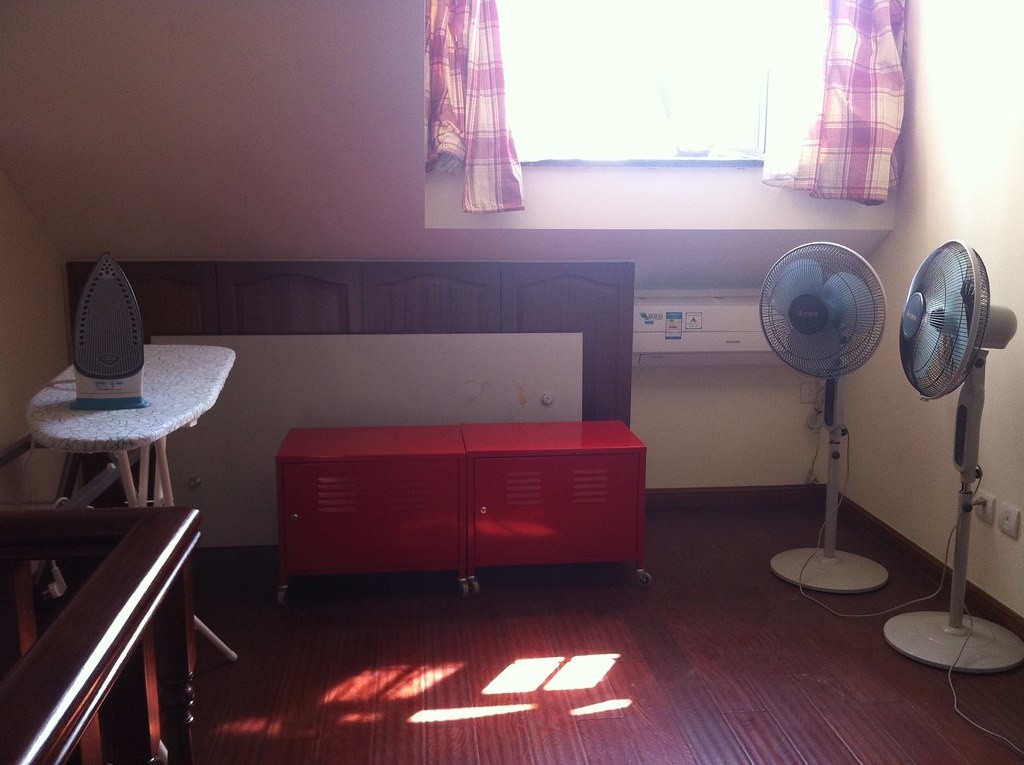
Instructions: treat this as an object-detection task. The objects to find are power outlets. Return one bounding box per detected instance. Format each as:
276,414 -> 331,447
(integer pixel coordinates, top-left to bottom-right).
973,488 -> 995,524
800,381 -> 817,404
998,503 -> 1021,540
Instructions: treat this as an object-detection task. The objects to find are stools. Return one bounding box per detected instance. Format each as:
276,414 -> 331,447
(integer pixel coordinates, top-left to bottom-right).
29,343 -> 238,662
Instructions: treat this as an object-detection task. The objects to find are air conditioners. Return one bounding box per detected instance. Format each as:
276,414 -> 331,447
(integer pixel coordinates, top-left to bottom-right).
631,288 -> 790,371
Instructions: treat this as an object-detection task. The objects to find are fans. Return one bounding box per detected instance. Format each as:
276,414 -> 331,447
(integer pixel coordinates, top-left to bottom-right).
757,243 -> 890,592
883,240 -> 1024,672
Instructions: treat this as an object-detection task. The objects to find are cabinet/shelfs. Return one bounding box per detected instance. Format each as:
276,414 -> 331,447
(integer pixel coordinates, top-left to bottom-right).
65,257 -> 637,425
276,426 -> 467,595
462,421 -> 653,590
0,507 -> 201,765
